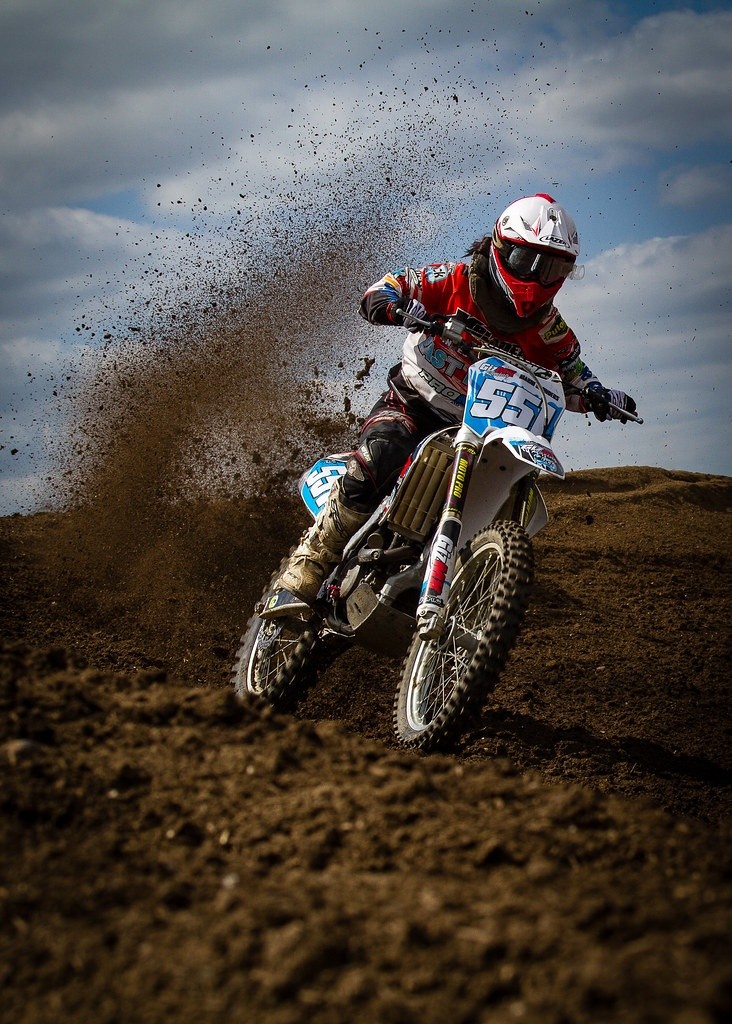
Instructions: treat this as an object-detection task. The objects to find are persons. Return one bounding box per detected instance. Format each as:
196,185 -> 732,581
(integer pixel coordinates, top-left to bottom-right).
257,194 -> 638,619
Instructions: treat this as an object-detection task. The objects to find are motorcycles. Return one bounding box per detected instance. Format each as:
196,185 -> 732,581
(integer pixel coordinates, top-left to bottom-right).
229,305 -> 644,750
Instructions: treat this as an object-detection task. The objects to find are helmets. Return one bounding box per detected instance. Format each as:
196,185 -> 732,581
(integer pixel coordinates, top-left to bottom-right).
489,194 -> 580,319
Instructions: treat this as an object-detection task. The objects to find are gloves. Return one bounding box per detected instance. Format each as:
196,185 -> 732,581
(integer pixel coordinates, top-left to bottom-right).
590,388 -> 638,424
390,297 -> 430,334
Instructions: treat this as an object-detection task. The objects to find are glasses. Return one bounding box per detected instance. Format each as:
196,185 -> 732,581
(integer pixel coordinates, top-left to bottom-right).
491,223 -> 586,283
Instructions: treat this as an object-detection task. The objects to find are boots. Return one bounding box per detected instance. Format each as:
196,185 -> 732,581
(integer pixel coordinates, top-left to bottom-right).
279,475 -> 378,606
